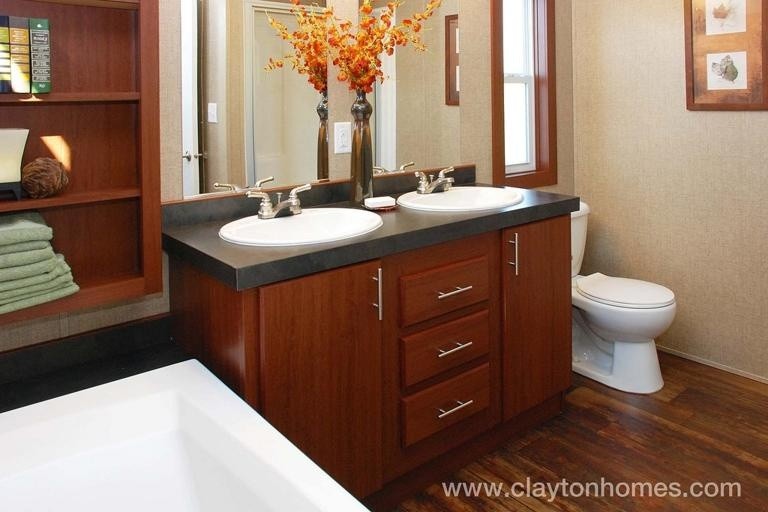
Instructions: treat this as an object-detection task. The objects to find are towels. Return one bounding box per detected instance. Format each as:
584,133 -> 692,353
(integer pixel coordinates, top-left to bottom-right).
0,212 -> 83,313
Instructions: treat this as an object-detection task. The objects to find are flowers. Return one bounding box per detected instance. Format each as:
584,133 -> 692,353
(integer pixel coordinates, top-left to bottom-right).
310,0 -> 447,92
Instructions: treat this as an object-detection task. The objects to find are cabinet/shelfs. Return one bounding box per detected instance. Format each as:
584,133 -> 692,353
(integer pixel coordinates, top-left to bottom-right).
389,230 -> 501,483
0,0 -> 164,326
166,256 -> 393,508
495,212 -> 574,429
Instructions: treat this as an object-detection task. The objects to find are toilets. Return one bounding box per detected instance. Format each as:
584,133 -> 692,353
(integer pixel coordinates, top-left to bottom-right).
570,200 -> 678,395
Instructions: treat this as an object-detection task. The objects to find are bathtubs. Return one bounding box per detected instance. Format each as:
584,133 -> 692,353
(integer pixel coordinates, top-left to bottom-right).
0,358 -> 372,512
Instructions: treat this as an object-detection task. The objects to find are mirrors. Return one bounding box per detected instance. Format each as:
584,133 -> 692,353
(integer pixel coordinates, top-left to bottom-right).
156,0 -> 331,210
356,0 -> 476,178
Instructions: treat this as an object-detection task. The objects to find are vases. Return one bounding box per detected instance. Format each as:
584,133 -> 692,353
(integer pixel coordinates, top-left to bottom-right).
314,95 -> 329,182
346,88 -> 377,206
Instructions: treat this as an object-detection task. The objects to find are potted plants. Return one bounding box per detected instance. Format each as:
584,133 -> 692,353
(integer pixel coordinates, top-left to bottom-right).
259,0 -> 337,94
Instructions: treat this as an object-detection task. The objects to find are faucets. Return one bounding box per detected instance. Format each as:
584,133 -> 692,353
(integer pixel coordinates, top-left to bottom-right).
273,199 -> 301,218
424,177 -> 455,194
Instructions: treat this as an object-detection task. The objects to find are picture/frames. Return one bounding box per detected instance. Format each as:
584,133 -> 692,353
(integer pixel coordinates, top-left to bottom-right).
682,0 -> 767,113
442,14 -> 460,106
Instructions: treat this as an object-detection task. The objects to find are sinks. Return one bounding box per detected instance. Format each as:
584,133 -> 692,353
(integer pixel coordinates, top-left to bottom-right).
218,207 -> 383,247
396,186 -> 524,212
